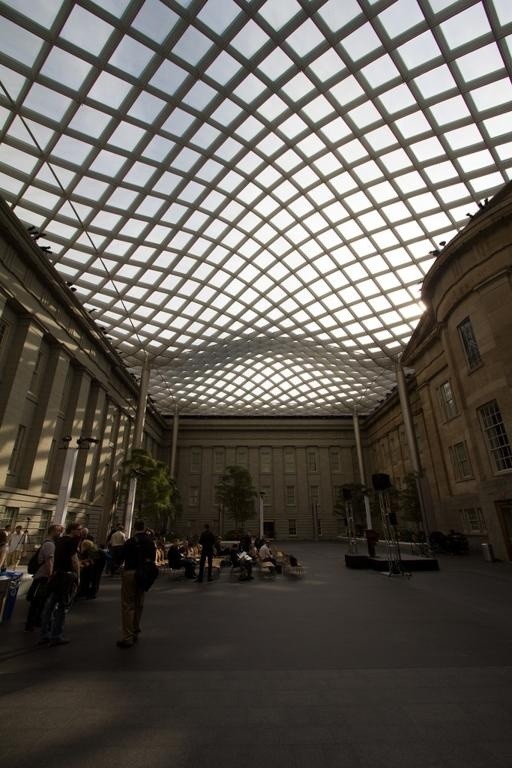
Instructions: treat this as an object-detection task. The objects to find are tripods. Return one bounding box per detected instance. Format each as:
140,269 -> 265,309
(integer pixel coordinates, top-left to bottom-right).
14,530 -> 33,558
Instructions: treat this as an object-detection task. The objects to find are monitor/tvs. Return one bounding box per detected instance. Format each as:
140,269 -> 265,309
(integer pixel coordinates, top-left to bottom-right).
342,488 -> 351,499
372,474 -> 390,490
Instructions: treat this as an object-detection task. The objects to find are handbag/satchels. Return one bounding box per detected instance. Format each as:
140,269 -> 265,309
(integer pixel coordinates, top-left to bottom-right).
134,560 -> 159,585
27,550 -> 41,574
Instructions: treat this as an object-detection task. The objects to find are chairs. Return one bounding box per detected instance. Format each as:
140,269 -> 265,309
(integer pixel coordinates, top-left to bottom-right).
153,539 -> 305,581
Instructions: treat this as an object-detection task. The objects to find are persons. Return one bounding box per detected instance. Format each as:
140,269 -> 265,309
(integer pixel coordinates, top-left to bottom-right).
0,512 -> 280,648
399,527 -> 469,556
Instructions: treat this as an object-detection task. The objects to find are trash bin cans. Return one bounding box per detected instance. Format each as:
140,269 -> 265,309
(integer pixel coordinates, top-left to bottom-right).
481,543 -> 495,562
0,572 -> 23,621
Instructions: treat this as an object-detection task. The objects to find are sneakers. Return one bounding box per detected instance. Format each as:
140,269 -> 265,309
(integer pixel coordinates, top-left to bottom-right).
25,621 -> 70,646
191,574 -> 213,583
116,636 -> 137,648
